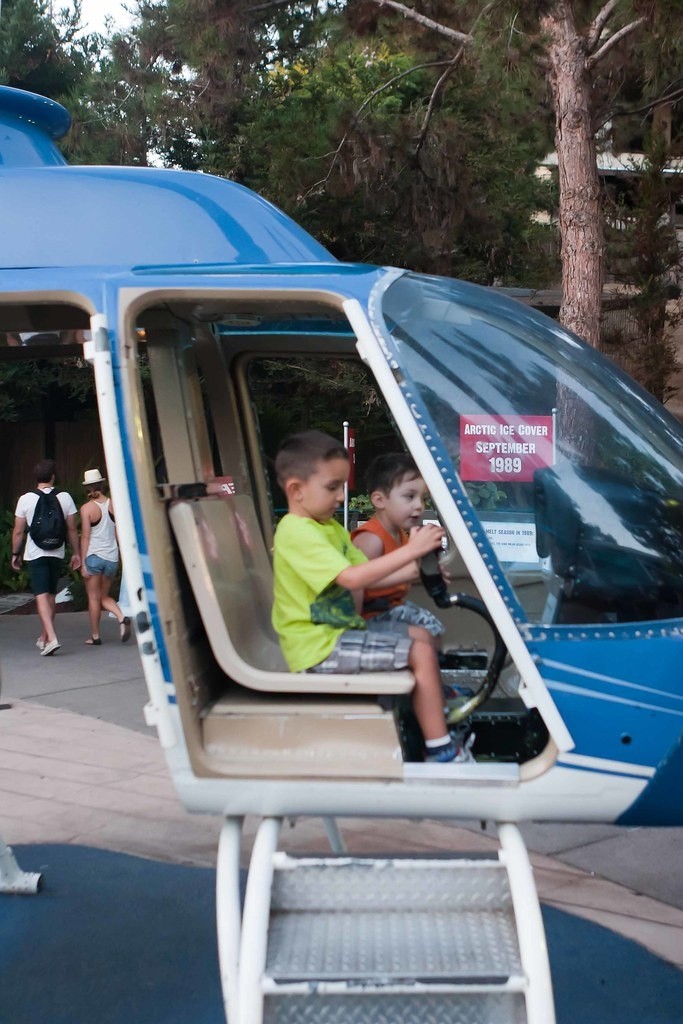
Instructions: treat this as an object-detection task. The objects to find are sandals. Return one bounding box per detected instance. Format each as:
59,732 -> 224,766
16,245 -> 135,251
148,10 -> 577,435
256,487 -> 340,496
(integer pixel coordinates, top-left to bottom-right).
84,635 -> 102,645
120,616 -> 131,642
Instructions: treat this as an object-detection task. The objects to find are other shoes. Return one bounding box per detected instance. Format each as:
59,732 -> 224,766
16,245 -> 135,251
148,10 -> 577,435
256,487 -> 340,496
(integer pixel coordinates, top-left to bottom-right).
425,741 -> 477,763
443,683 -> 475,700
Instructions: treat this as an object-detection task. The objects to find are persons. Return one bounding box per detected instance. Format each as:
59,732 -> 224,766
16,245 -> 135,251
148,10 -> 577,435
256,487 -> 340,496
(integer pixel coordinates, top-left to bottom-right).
11,459 -> 81,656
271,429 -> 476,764
349,453 -> 451,652
79,470 -> 131,646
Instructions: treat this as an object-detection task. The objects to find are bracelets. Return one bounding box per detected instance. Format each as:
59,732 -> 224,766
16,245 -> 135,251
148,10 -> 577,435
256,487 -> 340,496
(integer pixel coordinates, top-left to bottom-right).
12,553 -> 20,556
81,565 -> 86,567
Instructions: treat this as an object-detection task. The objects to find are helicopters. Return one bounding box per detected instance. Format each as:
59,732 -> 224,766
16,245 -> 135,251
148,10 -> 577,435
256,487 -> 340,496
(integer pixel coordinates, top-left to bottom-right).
0,85 -> 683,1024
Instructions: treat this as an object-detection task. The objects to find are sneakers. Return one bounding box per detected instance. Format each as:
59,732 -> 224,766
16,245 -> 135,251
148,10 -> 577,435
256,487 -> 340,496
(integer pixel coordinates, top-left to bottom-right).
40,640 -> 61,656
35,642 -> 45,649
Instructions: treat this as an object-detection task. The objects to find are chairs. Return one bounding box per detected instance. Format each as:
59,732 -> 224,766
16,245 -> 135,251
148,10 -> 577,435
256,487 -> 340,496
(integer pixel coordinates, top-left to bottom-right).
170,494 -> 415,697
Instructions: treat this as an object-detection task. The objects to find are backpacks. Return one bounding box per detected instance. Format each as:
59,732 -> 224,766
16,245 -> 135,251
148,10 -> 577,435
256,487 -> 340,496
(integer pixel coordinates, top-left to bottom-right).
27,488 -> 66,550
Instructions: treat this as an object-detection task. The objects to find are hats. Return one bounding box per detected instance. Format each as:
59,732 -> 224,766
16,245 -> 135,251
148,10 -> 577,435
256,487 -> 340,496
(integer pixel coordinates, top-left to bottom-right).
82,469 -> 106,485
